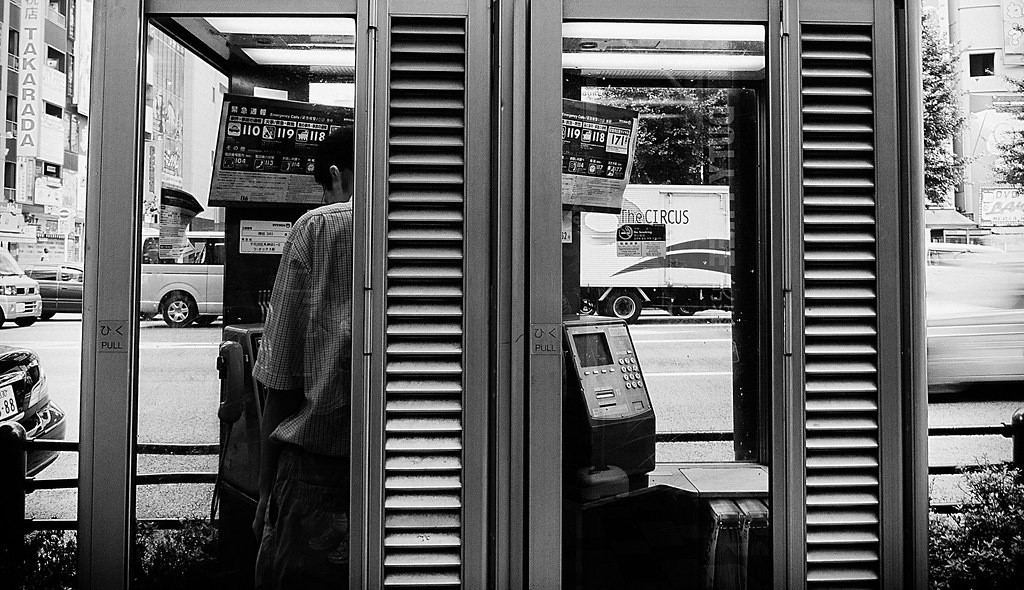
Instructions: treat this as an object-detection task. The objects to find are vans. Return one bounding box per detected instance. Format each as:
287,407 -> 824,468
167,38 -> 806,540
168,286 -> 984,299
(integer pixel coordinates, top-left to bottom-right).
140,230 -> 225,329
0,244 -> 43,326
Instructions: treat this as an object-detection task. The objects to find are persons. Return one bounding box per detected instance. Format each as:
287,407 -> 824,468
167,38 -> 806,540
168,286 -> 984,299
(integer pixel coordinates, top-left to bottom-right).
251,128 -> 355,590
41,248 -> 51,263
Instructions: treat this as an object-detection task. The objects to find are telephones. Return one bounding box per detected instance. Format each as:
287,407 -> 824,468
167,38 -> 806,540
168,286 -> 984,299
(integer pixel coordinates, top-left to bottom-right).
218,323 -> 269,494
561,315 -> 656,474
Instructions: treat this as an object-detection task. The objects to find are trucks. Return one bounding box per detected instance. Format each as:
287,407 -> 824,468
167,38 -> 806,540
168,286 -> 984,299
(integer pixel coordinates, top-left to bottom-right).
575,183 -> 732,325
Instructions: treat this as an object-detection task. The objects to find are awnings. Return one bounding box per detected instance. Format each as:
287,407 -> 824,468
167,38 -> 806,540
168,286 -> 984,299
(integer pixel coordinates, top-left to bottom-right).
925,210 -> 976,230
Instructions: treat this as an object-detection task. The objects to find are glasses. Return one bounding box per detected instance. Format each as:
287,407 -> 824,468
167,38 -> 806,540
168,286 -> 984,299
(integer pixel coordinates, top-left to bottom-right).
321,175 -> 332,204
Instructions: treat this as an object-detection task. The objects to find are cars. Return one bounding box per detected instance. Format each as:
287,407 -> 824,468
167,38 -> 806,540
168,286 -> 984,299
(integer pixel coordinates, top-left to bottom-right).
25,265 -> 83,320
0,344 -> 67,478
924,243 -> 1024,389
